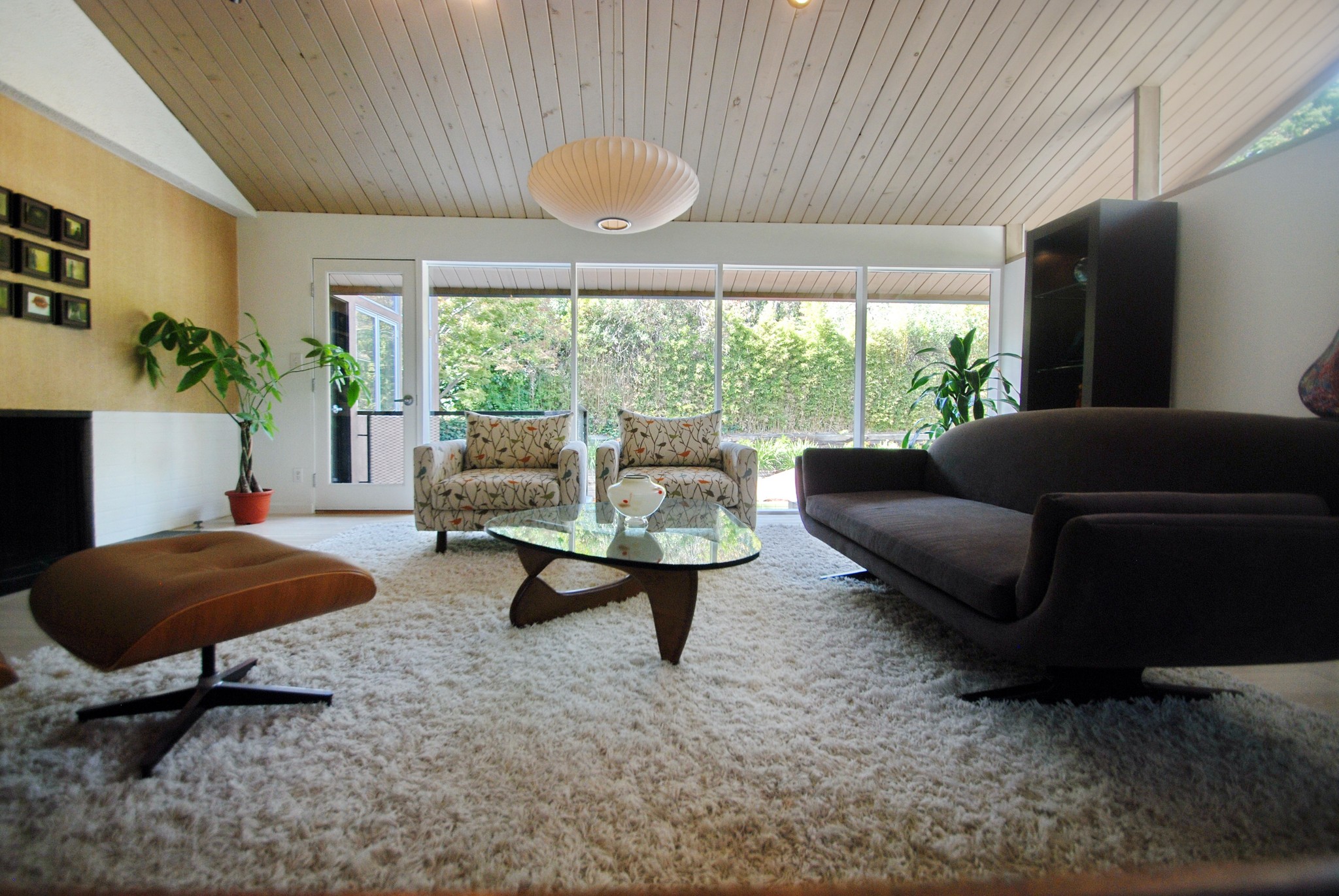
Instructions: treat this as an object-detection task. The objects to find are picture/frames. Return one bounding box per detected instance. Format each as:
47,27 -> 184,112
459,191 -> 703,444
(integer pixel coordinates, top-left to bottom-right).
0,185 -> 93,333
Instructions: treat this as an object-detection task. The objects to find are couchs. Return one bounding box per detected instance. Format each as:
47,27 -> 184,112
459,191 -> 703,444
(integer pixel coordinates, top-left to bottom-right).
596,408 -> 759,534
794,408 -> 1290,704
414,409 -> 586,554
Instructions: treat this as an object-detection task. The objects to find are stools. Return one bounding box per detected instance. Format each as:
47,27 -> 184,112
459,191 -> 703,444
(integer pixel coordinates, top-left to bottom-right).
24,529 -> 377,779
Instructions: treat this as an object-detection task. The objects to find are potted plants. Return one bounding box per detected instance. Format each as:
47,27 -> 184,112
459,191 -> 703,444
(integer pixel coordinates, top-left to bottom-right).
135,310 -> 371,525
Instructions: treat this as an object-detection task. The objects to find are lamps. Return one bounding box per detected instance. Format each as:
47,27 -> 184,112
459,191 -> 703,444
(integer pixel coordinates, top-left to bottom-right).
525,0 -> 701,236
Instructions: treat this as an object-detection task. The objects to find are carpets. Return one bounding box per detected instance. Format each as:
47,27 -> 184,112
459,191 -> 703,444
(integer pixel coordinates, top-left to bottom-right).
0,520 -> 1339,896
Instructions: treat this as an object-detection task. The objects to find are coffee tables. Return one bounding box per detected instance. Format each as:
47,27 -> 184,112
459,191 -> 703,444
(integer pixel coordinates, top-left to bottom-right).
484,495 -> 761,669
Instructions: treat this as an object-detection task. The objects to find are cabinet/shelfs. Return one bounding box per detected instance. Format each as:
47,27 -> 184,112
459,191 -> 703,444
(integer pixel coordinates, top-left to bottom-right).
1018,199 -> 1176,411
0,408 -> 242,601
